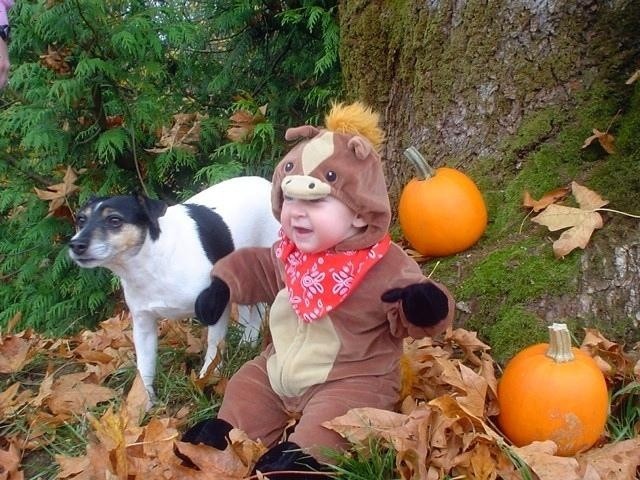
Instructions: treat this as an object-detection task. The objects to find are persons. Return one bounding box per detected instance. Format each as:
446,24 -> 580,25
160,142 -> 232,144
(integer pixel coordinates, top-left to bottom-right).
174,97 -> 457,480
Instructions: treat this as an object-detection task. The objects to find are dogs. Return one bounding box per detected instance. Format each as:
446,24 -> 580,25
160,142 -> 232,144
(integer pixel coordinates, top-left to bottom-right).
67,177 -> 281,423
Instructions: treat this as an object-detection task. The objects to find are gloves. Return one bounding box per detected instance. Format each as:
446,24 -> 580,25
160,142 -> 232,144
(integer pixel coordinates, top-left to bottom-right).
380,279 -> 450,326
192,277 -> 231,326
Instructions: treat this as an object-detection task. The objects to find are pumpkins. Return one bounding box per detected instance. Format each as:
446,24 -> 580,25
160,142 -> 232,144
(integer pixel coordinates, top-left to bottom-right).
398,145 -> 489,257
496,322 -> 609,457
